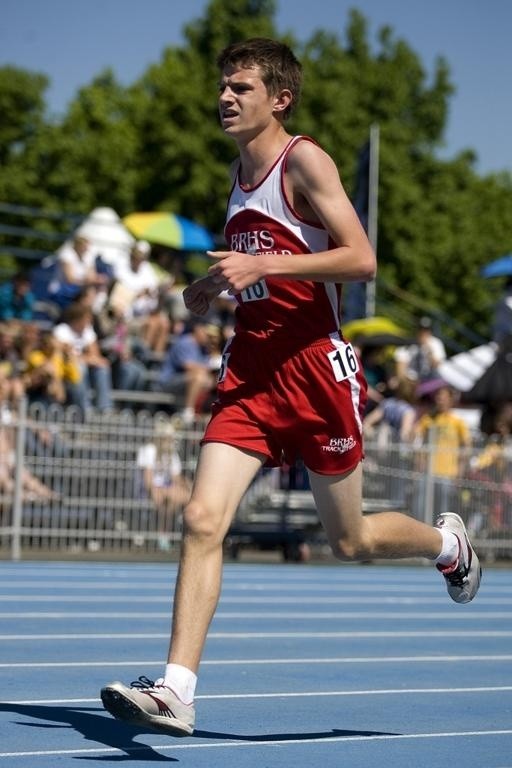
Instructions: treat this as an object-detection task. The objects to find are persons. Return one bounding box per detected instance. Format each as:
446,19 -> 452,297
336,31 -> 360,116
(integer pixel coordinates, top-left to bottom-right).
1,238 -> 239,549
99,36 -> 482,736
240,273 -> 512,549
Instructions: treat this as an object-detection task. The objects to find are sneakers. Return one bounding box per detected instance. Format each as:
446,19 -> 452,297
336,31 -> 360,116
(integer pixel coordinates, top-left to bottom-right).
432,512 -> 482,604
100,675 -> 197,738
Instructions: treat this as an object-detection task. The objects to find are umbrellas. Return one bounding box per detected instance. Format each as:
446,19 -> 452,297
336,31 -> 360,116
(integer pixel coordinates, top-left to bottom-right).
121,210 -> 216,252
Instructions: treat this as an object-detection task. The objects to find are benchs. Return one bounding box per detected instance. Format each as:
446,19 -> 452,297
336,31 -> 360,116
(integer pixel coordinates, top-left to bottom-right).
0,376 -> 325,559
